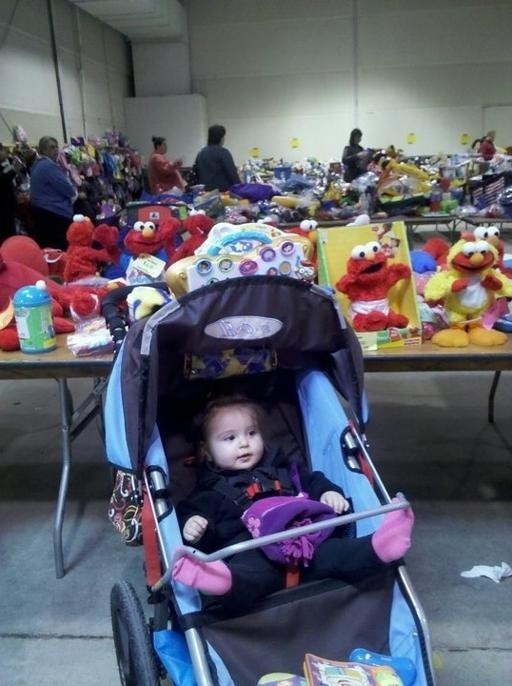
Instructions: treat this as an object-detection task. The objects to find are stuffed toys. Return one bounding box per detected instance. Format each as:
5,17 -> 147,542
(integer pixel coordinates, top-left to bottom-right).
284,218 -> 322,267
333,239 -> 411,330
407,216 -> 511,350
0,209 -> 218,353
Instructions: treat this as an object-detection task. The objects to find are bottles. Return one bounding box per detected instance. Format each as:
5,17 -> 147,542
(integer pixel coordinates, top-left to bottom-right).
12,280 -> 60,354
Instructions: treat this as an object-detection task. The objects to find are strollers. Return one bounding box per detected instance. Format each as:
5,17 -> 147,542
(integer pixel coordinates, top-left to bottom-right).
102,276 -> 436,686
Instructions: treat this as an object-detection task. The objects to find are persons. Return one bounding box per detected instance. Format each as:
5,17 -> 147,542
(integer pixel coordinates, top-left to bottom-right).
24,135 -> 79,253
478,130 -> 497,161
161,386 -> 416,608
146,134 -> 186,194
195,124 -> 244,190
341,127 -> 374,183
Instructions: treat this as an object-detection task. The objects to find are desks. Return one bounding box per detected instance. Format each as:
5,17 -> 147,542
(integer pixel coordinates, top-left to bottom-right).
0,307 -> 511,577
279,217 -> 511,251
